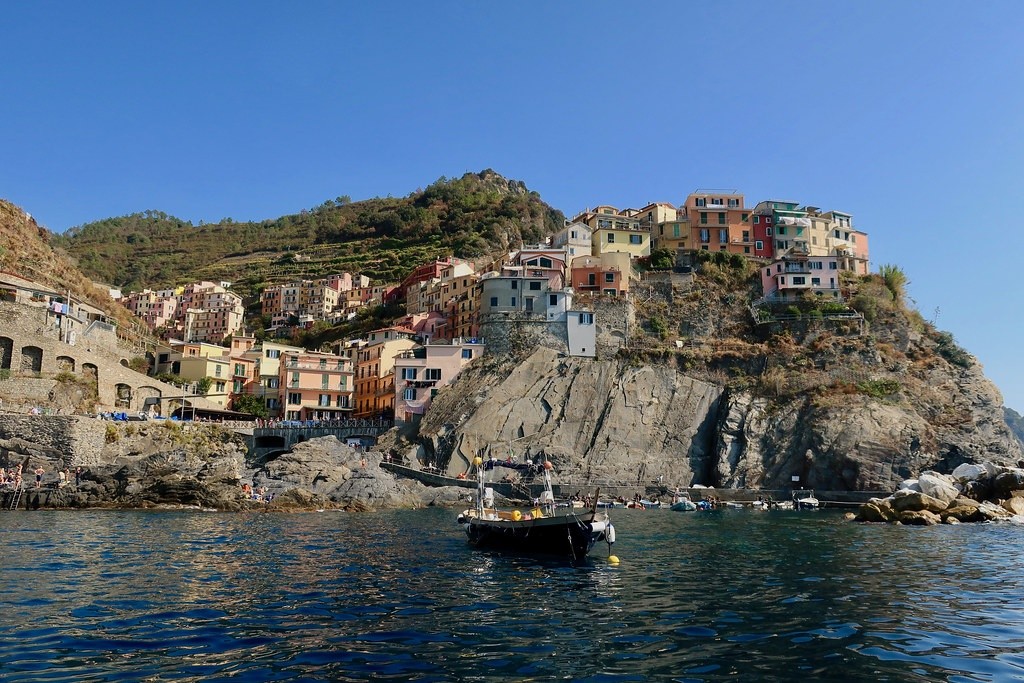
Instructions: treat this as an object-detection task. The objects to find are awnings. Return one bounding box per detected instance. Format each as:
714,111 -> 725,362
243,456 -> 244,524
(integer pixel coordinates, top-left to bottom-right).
305,405 -> 353,413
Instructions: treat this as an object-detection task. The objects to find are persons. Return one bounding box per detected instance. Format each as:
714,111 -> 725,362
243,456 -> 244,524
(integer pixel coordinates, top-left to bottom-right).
707,496 -> 719,505
255,417 -> 279,428
0,464 -> 22,492
350,442 -> 360,447
656,475 -> 663,482
242,484 -> 275,503
635,491 -> 642,502
362,458 -> 365,466
195,415 -> 198,421
575,490 -> 591,508
103,410 -> 129,421
59,468 -> 69,483
386,452 -> 391,463
675,486 -> 678,494
35,466 -> 45,489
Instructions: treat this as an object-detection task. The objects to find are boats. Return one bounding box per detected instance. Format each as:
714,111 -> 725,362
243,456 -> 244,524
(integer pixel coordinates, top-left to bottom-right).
457,514 -> 610,556
504,493 -> 820,511
